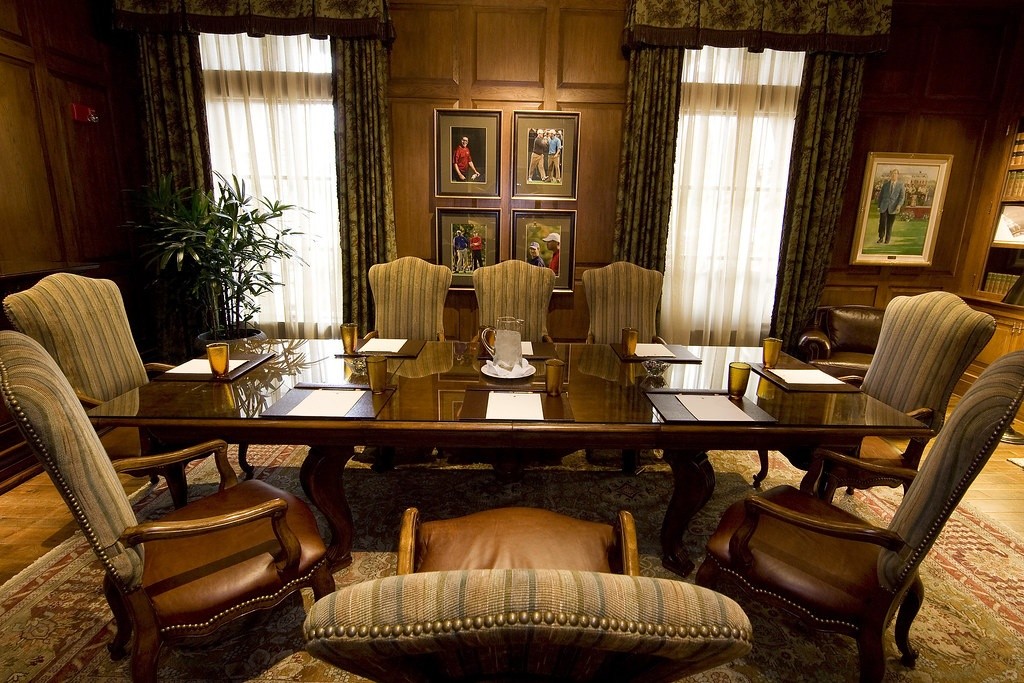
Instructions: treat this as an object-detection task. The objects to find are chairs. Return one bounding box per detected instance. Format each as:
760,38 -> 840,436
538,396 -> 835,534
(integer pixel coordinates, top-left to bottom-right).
362,256 -> 452,342
753,290 -> 997,503
696,350 -> 1024,682
797,305 -> 885,388
472,259 -> 555,342
0,329 -> 336,682
2,273 -> 254,511
582,261 -> 667,344
301,507 -> 753,683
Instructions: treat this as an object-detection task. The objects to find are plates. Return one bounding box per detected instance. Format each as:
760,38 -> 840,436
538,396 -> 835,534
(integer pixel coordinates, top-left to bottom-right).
481,364 -> 536,378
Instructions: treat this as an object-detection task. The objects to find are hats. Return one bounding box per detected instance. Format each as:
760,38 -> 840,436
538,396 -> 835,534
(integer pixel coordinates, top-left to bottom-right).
530,242 -> 540,249
549,129 -> 555,134
542,232 -> 560,243
537,129 -> 545,134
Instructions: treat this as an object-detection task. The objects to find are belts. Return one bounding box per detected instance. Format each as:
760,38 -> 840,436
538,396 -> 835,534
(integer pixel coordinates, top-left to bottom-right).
534,151 -> 544,155
549,154 -> 555,155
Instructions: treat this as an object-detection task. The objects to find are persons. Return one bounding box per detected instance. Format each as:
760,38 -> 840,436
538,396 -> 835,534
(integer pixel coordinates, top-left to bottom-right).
528,128 -> 563,184
452,137 -> 480,180
527,233 -> 559,276
877,169 -> 905,244
453,229 -> 484,273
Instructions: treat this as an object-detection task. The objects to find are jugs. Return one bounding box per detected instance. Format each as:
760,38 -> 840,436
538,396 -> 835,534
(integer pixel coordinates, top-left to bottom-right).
481,317 -> 524,372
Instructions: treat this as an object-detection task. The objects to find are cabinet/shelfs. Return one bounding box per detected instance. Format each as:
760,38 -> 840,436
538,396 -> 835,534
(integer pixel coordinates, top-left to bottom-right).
952,116 -> 1024,437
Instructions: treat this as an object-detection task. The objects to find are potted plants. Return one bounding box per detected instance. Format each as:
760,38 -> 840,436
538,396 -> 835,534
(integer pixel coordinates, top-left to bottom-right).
117,166 -> 304,353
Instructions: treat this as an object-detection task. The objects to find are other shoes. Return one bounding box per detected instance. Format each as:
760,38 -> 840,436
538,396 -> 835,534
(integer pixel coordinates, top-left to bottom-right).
529,177 -> 533,182
885,240 -> 889,244
877,237 -> 883,243
454,271 -> 459,273
542,176 -> 550,182
556,179 -> 560,183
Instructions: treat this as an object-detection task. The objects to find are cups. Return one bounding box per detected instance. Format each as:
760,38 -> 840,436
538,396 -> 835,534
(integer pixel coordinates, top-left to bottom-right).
546,359 -> 566,395
340,323 -> 358,354
365,355 -> 387,394
763,338 -> 782,368
480,325 -> 496,356
622,328 -> 638,355
728,362 -> 750,399
206,342 -> 229,378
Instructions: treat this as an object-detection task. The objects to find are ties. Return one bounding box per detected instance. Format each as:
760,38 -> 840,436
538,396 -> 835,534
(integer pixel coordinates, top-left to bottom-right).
891,182 -> 894,193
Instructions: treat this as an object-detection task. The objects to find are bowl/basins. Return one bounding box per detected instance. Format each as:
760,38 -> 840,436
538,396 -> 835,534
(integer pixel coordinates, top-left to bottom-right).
640,361 -> 670,377
343,357 -> 367,374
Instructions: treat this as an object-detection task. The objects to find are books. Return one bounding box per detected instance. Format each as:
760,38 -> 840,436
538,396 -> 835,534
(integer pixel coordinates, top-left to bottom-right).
985,272 -> 1024,306
1004,132 -> 1024,202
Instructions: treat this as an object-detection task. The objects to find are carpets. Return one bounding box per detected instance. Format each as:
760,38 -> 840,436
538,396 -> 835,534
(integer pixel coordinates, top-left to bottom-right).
0,442 -> 1024,683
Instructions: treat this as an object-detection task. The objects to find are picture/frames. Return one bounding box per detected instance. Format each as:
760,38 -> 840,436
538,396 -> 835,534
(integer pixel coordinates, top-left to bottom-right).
437,389 -> 466,421
434,108 -> 503,199
848,152 -> 954,268
511,110 -> 581,201
437,343 -> 572,384
510,208 -> 577,293
435,206 -> 502,290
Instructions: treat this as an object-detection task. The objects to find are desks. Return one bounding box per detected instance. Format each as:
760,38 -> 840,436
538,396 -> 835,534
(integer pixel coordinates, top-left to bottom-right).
87,339 -> 936,574
